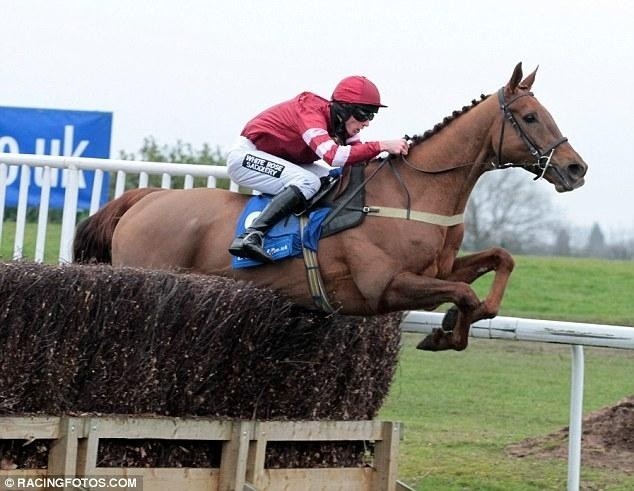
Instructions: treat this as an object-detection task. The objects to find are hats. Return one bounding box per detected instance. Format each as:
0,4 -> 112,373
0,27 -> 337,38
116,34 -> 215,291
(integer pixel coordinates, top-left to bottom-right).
331,75 -> 388,108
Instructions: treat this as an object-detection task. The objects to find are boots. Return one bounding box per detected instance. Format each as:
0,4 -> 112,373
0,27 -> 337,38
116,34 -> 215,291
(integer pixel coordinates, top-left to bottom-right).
227,184 -> 308,265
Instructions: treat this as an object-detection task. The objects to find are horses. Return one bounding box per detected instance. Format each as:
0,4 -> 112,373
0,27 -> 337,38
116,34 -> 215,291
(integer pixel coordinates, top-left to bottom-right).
70,60 -> 589,353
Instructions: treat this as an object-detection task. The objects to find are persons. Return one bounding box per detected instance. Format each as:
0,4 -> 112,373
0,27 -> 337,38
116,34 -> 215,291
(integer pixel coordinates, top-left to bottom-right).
228,76 -> 411,264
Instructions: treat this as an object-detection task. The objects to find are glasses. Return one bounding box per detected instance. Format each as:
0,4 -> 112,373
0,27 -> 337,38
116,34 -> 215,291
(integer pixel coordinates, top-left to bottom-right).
339,103 -> 374,122
